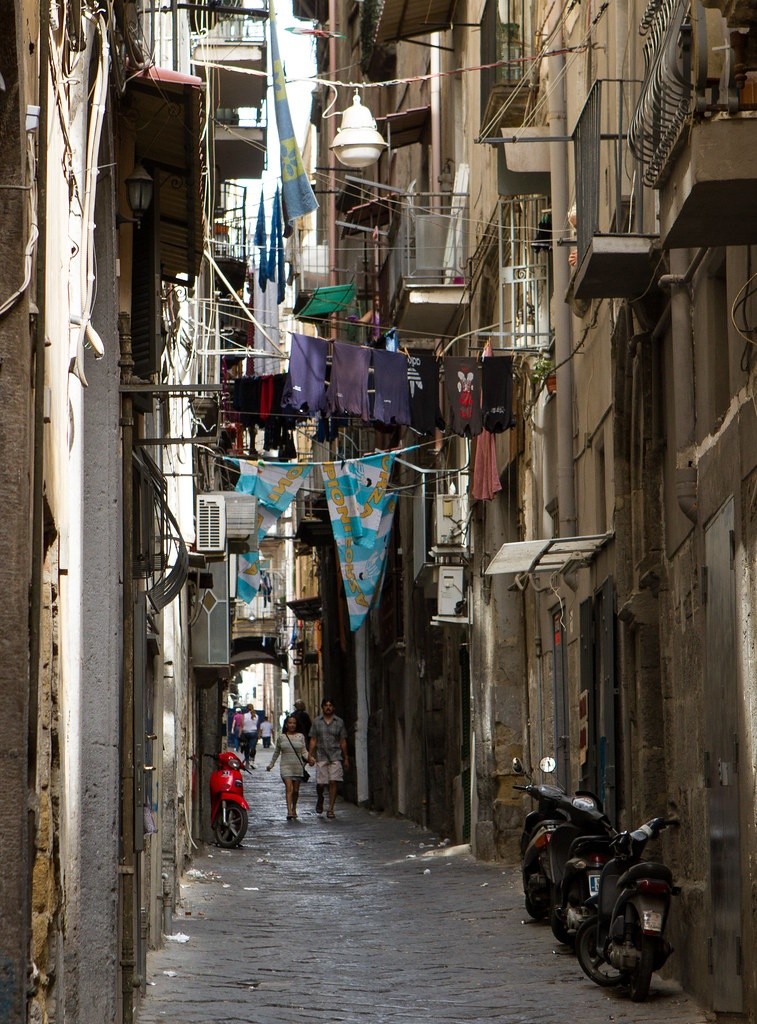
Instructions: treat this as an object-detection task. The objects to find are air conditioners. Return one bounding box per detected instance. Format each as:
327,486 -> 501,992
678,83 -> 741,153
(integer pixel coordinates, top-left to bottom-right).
431,493 -> 462,546
195,495 -> 228,552
437,566 -> 463,616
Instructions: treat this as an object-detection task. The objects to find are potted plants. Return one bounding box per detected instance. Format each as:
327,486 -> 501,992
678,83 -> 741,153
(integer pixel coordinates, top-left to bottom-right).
530,356 -> 556,395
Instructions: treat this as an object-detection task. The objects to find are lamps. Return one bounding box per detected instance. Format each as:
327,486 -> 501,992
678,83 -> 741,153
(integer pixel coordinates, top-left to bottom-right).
329,89 -> 391,170
116,160 -> 153,230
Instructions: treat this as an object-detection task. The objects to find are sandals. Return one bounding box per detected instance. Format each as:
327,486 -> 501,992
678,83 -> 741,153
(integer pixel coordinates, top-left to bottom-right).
327,811 -> 335,818
316,797 -> 324,813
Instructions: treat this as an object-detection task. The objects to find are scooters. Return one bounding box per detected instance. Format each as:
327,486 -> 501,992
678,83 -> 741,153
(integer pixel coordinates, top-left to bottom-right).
510,756 -> 683,1003
204,749 -> 256,850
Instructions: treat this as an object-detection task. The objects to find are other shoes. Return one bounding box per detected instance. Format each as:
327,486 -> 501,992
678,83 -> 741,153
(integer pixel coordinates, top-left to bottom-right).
292,809 -> 297,818
287,815 -> 294,820
250,763 -> 257,769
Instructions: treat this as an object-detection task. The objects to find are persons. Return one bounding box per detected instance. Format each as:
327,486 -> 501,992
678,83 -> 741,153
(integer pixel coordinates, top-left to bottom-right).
282,699 -> 312,782
307,698 -> 350,817
266,715 -> 316,818
238,704 -> 259,768
259,717 -> 273,748
231,708 -> 243,751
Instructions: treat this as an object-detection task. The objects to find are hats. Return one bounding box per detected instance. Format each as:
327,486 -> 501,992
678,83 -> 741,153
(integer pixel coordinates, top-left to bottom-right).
236,708 -> 241,712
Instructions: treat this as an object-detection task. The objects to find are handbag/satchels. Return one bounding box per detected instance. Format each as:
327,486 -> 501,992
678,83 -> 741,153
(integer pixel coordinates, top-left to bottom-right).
302,771 -> 310,783
